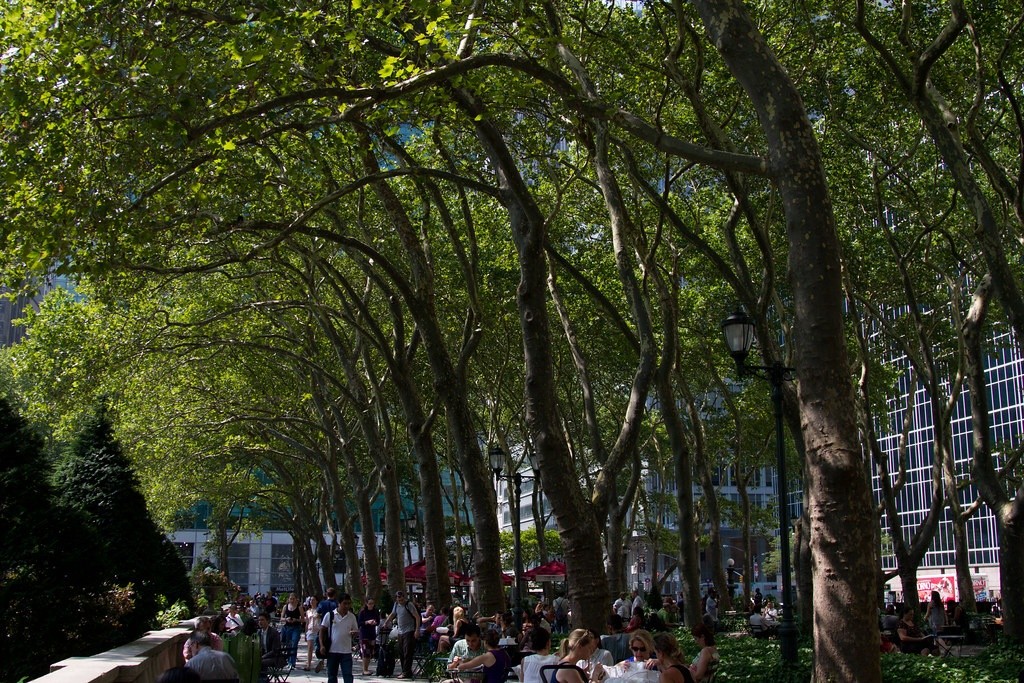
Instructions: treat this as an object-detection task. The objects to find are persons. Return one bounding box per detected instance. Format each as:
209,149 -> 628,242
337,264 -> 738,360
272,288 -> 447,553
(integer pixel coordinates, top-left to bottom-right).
439,625 -> 487,683
303,595 -> 324,673
520,627 -> 560,683
162,586 -> 949,683
382,591 -> 420,678
280,593 -> 304,669
575,629 -> 614,675
687,625 -> 720,683
653,633 -> 695,683
258,614 -> 283,683
599,614 -> 633,665
550,629 -> 604,683
320,592 -> 360,683
357,596 -> 379,675
184,631 -> 239,679
613,628 -> 662,673
183,617 -> 224,662
316,588 -> 338,624
457,629 -> 510,683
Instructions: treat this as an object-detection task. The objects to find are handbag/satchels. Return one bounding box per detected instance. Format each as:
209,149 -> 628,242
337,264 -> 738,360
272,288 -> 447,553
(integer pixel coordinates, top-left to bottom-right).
555,599 -> 567,624
395,601 -> 422,628
316,612 -> 334,659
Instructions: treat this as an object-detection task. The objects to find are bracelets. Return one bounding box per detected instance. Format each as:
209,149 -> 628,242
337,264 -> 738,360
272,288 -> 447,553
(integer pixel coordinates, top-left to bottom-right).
416,630 -> 420,631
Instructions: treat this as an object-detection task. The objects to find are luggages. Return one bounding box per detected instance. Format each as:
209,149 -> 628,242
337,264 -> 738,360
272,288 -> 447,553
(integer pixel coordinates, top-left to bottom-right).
376,627 -> 395,678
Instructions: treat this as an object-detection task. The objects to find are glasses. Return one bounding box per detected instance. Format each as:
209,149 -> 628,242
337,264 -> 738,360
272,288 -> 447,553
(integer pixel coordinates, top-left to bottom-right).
631,647 -> 646,652
572,630 -> 592,651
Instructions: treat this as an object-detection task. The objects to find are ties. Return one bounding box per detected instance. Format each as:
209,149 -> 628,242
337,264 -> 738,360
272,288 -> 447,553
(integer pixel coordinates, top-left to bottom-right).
261,632 -> 264,649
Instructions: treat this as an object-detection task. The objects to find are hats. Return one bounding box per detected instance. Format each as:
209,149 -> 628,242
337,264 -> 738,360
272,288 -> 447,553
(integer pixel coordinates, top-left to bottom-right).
531,628 -> 549,649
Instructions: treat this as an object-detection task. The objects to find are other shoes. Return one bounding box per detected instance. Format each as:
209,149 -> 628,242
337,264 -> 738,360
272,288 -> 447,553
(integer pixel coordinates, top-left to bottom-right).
397,673 -> 413,679
302,668 -> 310,671
286,664 -> 295,670
361,670 -> 373,676
315,661 -> 323,673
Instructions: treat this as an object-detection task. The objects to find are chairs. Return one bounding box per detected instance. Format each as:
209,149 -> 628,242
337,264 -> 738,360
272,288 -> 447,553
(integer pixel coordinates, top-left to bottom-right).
258,616 -> 486,683
933,625 -> 961,656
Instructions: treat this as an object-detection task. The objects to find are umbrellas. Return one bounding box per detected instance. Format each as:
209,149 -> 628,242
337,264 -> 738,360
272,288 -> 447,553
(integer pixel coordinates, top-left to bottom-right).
358,560 -> 574,586
